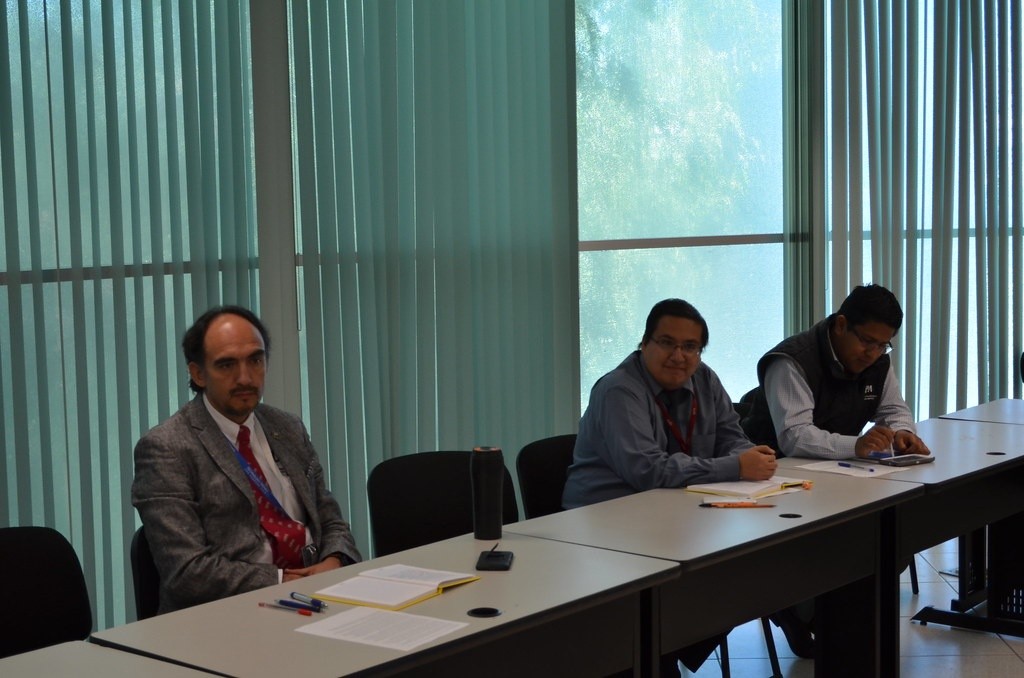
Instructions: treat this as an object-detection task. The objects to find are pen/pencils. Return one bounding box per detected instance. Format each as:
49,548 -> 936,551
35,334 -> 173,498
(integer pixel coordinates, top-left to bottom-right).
698,501 -> 757,508
275,599 -> 328,612
884,416 -> 895,457
837,462 -> 875,472
290,592 -> 329,608
717,504 -> 777,508
258,602 -> 313,616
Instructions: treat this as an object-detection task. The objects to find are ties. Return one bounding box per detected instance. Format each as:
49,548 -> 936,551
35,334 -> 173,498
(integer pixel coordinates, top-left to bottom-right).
236,425 -> 307,570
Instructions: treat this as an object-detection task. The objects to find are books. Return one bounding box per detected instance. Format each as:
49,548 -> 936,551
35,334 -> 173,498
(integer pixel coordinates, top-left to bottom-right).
686,475 -> 812,498
312,564 -> 479,609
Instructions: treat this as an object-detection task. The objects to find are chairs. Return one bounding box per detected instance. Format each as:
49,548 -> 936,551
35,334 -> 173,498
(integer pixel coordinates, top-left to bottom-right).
517,433 -> 729,677
368,449 -> 520,560
730,386 -> 785,677
131,524 -> 160,621
0,525 -> 92,659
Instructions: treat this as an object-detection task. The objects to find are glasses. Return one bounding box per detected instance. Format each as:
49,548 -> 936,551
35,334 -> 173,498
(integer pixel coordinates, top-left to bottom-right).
845,322 -> 894,355
648,337 -> 702,357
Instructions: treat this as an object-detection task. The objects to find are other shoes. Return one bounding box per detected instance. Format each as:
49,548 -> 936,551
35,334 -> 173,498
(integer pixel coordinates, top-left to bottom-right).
770,609 -> 815,659
659,652 -> 681,678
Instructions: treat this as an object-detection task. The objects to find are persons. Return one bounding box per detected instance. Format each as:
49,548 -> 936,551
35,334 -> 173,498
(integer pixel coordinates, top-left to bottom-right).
562,299 -> 778,678
742,284 -> 930,660
130,306 -> 364,616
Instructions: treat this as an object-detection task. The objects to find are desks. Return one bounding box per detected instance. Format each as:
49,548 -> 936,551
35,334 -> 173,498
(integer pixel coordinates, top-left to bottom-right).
0,396 -> 1023,678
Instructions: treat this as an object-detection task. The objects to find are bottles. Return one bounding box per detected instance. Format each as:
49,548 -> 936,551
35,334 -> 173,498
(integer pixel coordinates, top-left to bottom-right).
471,446 -> 505,541
